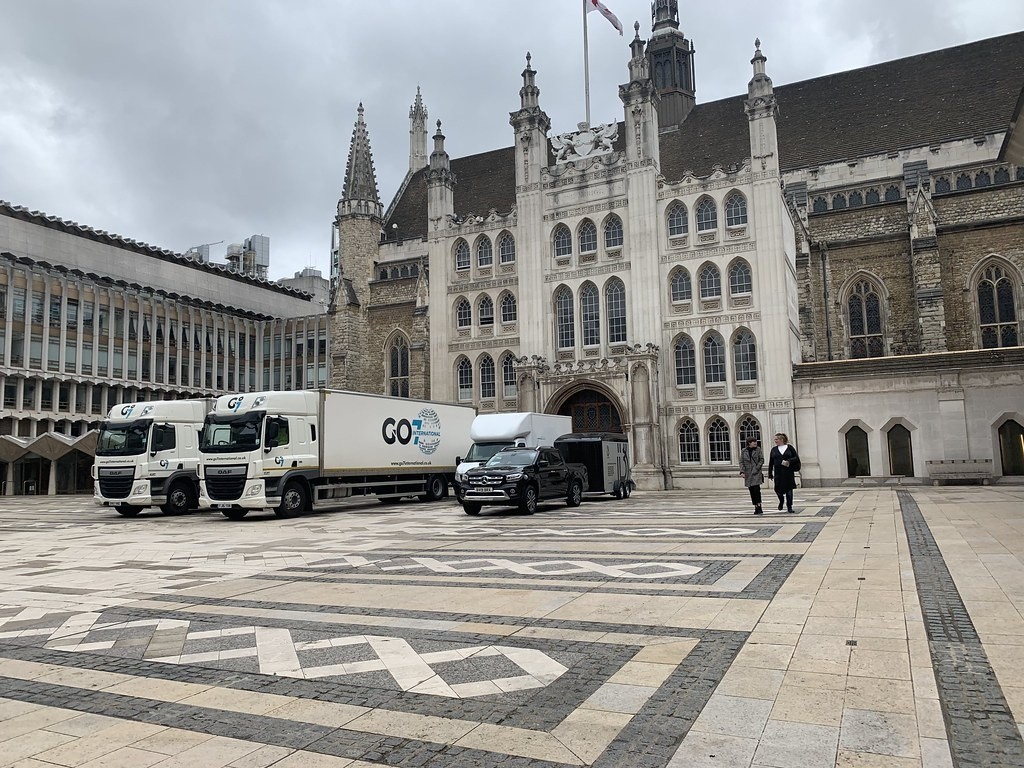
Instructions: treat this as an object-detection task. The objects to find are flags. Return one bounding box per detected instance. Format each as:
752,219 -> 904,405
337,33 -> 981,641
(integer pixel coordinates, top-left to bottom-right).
586,0 -> 622,36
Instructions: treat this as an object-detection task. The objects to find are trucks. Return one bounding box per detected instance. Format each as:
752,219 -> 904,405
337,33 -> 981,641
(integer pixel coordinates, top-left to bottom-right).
452,412 -> 573,503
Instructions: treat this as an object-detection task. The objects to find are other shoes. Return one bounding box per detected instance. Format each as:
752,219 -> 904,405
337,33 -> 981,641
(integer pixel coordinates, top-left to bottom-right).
786,504 -> 795,512
755,506 -> 762,515
778,501 -> 783,510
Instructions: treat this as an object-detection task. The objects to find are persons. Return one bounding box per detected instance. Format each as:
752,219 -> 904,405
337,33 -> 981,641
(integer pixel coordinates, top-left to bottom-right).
739,437 -> 764,514
768,433 -> 800,512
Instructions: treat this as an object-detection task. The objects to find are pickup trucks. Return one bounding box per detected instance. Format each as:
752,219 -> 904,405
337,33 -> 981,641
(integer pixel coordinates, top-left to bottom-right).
456,445 -> 589,514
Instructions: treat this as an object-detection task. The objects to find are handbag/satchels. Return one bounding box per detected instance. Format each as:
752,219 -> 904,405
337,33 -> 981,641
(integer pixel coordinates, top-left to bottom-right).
793,460 -> 801,471
761,473 -> 764,482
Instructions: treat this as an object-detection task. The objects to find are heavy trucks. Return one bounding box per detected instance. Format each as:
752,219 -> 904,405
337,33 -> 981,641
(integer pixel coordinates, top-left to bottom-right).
197,388 -> 479,518
90,399 -> 232,516
